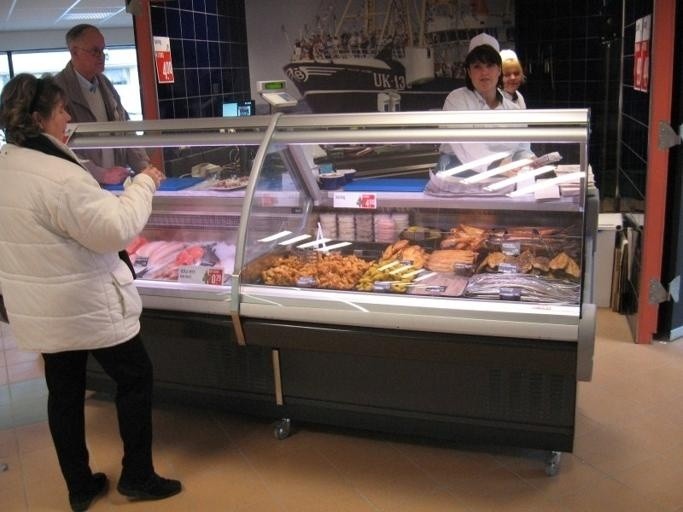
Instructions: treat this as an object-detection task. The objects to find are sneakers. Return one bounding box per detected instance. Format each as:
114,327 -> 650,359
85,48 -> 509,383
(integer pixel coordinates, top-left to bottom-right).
69,472 -> 107,512
117,467 -> 182,499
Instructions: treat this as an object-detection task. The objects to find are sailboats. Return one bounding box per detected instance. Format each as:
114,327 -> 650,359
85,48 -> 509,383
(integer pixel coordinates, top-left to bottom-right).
282,1 -> 516,182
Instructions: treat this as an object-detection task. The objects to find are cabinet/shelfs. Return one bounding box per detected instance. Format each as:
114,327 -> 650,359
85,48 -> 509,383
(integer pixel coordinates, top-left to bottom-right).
61,107 -> 601,476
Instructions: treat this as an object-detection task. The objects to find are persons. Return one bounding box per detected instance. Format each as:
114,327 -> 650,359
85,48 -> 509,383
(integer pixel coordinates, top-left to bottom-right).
1,72 -> 183,511
52,24 -> 154,188
498,48 -> 540,163
437,33 -> 541,178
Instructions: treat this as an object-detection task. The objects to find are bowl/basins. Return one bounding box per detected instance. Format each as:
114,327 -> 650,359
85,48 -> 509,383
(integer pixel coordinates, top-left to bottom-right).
399,226 -> 446,250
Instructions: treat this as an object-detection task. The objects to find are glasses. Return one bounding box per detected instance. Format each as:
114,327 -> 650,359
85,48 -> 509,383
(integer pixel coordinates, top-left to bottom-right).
73,46 -> 104,57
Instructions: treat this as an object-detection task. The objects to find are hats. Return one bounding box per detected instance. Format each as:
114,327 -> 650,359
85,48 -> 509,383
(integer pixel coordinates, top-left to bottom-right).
498,49 -> 520,65
466,32 -> 500,55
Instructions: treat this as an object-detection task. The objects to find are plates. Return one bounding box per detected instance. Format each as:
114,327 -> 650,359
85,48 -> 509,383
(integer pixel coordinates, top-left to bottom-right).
208,181 -> 249,192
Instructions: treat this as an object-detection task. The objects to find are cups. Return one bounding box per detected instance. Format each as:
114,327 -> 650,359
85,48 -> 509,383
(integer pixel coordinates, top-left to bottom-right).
318,169 -> 356,191
